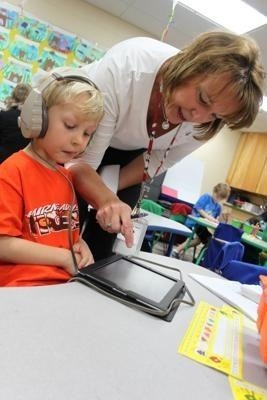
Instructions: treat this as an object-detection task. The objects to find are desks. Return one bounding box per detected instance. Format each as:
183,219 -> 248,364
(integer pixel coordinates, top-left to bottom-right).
0,250 -> 267,349
137,203 -> 192,257
178,214 -> 266,266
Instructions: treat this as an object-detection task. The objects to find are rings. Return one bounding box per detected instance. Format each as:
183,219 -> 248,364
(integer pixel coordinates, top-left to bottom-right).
107,223 -> 112,228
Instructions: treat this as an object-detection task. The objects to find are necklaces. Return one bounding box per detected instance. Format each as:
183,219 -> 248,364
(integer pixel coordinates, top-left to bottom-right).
160,104 -> 169,131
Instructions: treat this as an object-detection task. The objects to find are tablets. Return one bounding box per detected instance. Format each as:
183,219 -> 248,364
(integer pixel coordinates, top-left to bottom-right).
79,253 -> 186,313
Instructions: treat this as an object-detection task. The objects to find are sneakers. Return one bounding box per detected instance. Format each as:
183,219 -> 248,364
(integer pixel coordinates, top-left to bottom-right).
172,248 -> 178,258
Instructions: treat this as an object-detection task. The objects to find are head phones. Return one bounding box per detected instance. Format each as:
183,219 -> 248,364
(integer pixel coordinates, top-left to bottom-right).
17,68 -> 105,159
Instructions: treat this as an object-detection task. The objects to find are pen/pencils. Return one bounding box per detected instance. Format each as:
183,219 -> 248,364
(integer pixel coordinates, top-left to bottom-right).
119,213 -> 148,224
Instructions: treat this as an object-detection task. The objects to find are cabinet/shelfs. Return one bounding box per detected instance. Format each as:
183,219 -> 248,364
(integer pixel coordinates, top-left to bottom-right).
222,132 -> 267,216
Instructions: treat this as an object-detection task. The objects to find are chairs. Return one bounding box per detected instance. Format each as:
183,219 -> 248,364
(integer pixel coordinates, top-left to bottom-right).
140,197 -> 166,217
216,241 -> 267,285
206,223 -> 244,273
152,201 -> 192,252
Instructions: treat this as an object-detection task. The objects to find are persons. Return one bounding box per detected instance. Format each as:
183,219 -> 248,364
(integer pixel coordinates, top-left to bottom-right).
62,31 -> 264,268
0,68 -> 104,289
0,83 -> 33,164
169,183 -> 231,260
